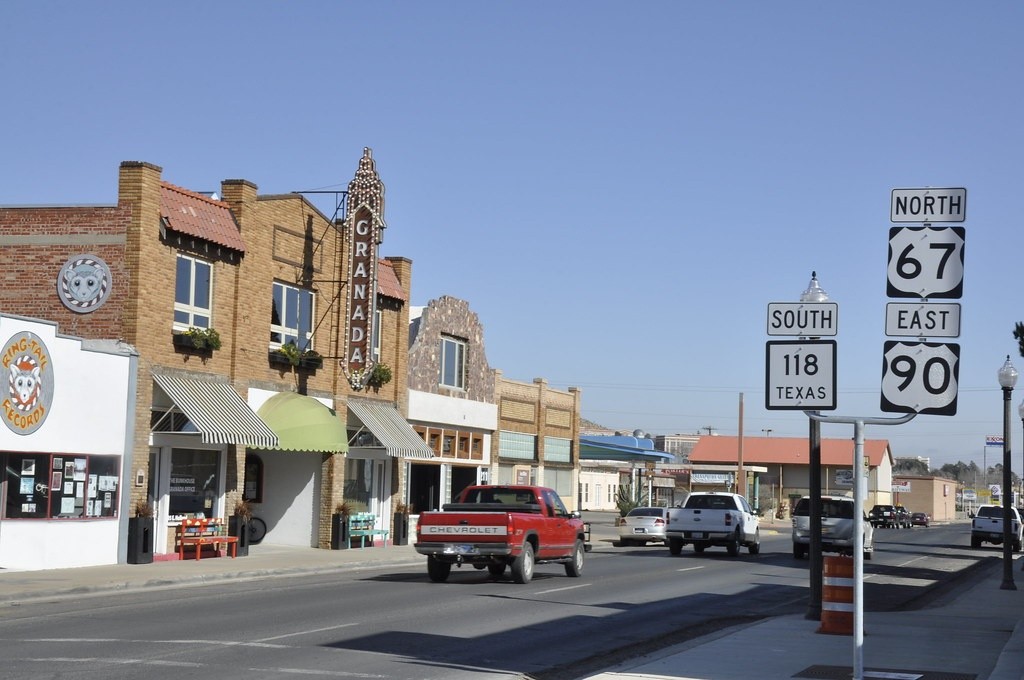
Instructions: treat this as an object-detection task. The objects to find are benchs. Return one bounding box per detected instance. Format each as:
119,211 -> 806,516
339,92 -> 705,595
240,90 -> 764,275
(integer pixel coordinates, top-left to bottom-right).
180,518 -> 238,560
347,514 -> 389,549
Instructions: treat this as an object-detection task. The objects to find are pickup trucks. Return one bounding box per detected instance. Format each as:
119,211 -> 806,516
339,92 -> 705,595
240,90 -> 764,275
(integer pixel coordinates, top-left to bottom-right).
970,505 -> 1024,551
414,485 -> 592,583
667,491 -> 760,555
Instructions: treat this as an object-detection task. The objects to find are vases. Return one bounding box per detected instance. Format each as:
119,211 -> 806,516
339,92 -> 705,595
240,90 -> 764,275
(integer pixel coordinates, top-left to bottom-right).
174,334 -> 212,351
269,352 -> 323,370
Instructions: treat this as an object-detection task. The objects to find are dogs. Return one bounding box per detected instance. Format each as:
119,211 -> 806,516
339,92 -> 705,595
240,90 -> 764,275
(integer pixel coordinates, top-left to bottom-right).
64,268 -> 104,303
9,363 -> 41,403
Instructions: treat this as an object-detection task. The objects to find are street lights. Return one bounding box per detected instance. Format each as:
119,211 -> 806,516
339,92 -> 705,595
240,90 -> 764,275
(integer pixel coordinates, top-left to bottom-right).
997,355 -> 1018,591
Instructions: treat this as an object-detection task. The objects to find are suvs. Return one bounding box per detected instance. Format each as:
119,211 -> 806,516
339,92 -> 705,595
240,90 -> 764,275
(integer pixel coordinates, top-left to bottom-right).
868,504 -> 900,528
894,507 -> 911,528
793,495 -> 874,561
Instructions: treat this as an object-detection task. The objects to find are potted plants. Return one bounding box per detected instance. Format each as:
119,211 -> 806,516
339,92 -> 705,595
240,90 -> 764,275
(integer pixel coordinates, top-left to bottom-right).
228,498 -> 252,557
127,497 -> 156,564
393,499 -> 414,546
332,500 -> 353,552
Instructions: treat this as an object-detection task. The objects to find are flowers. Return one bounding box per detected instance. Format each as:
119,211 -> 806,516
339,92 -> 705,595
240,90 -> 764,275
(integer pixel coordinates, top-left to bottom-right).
274,343 -> 323,368
372,362 -> 392,384
179,326 -> 223,350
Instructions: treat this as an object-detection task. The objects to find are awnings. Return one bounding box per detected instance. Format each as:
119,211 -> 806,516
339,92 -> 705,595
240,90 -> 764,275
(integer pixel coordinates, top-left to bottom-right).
249,391 -> 350,454
347,402 -> 435,459
151,372 -> 280,448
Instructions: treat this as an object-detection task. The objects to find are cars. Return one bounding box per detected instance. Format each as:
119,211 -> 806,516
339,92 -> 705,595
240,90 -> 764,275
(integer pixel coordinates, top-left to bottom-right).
912,513 -> 930,527
620,507 -> 669,545
